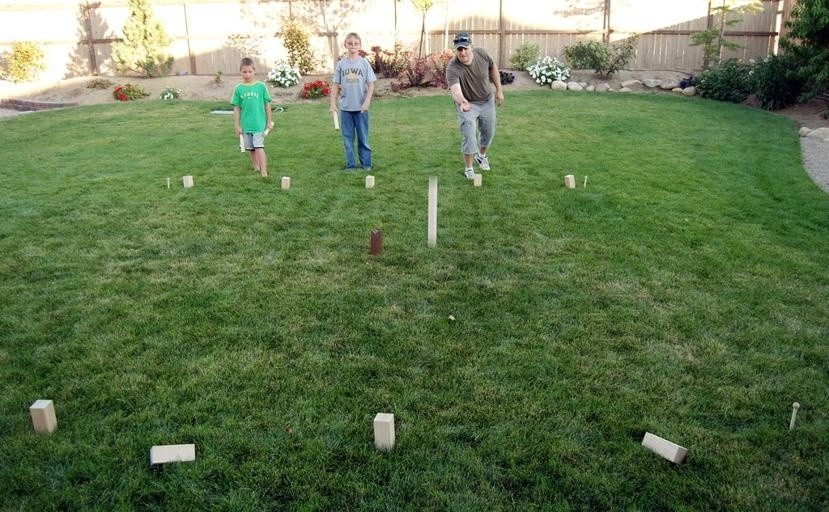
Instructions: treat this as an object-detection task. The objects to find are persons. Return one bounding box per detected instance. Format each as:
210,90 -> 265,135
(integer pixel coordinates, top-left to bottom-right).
229,58 -> 273,178
330,32 -> 377,172
445,33 -> 504,180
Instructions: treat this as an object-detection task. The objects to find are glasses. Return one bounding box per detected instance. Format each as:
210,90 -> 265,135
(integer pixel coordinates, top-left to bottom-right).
452,36 -> 470,44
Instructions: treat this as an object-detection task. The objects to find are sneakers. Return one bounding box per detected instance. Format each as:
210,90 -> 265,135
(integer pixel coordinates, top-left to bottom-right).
463,165 -> 475,180
473,152 -> 493,170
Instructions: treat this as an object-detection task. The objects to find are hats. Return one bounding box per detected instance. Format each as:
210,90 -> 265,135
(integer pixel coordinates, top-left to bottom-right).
451,31 -> 472,51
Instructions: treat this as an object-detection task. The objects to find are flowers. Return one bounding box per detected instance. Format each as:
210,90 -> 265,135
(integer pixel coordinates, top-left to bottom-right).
158,86 -> 181,101
266,64 -> 302,89
526,56 -> 572,85
302,80 -> 330,100
115,82 -> 148,101
